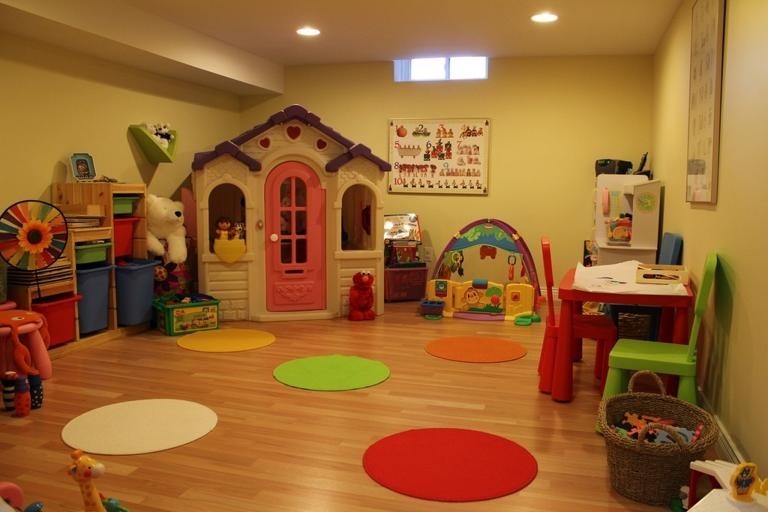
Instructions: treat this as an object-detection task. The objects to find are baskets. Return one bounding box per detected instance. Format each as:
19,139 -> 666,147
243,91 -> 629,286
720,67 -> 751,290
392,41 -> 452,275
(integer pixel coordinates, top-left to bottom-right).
597,368 -> 721,505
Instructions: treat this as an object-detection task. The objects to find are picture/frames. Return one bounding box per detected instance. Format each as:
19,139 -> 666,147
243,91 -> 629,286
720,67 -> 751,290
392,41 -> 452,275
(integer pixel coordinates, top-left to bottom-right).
684,0 -> 725,205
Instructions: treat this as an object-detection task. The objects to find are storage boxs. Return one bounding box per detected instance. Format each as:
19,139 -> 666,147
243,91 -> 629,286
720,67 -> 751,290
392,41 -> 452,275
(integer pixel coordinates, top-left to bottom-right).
156,292 -> 221,336
384,266 -> 429,303
115,257 -> 160,329
112,217 -> 141,256
30,292 -> 81,348
76,267 -> 116,336
74,240 -> 112,267
113,193 -> 140,215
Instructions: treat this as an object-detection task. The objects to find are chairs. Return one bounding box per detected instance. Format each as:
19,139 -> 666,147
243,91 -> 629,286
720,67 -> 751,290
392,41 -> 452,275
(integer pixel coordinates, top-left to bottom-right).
538,236 -> 618,401
593,251 -> 721,439
604,231 -> 682,339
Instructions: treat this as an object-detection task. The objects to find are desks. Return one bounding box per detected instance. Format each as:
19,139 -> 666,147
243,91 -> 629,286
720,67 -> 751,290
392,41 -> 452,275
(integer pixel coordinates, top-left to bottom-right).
0,309 -> 54,418
549,267 -> 695,401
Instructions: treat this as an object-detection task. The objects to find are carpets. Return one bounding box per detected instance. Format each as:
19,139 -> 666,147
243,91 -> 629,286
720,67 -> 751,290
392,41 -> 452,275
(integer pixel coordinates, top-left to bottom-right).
273,353 -> 392,390
176,325 -> 277,354
60,398 -> 218,456
362,427 -> 538,502
423,336 -> 527,364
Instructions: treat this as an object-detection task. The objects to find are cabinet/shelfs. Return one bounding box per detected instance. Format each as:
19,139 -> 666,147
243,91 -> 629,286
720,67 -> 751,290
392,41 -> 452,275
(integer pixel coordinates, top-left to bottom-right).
593,176 -> 660,266
12,182 -> 147,364
